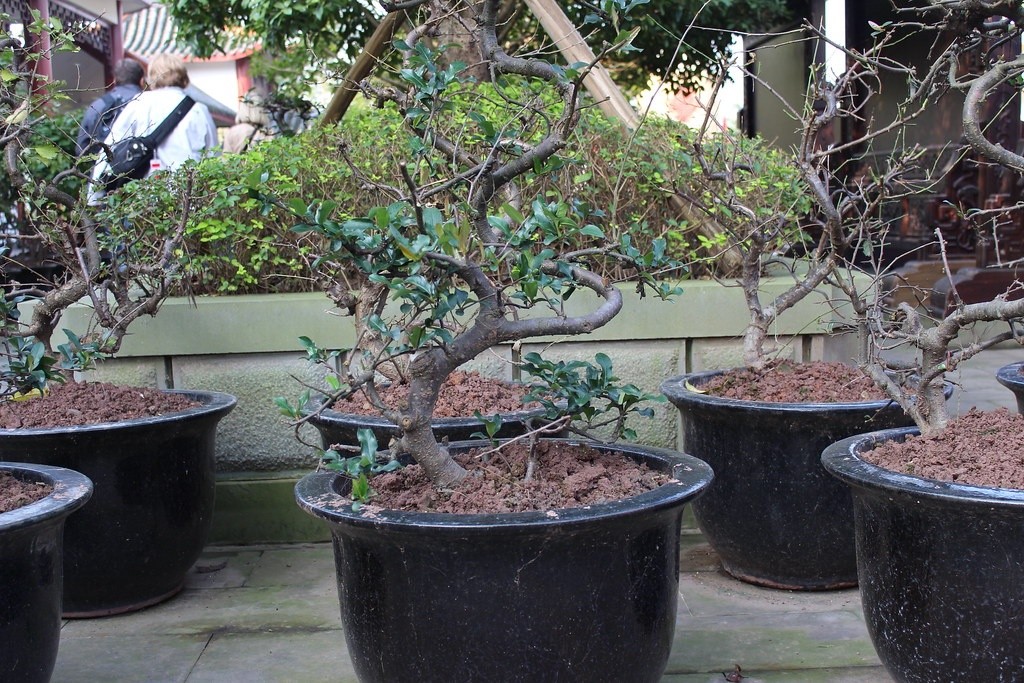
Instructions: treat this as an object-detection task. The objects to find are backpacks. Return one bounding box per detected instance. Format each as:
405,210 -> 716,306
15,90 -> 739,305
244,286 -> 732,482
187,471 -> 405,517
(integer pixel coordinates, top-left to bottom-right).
93,92 -> 127,142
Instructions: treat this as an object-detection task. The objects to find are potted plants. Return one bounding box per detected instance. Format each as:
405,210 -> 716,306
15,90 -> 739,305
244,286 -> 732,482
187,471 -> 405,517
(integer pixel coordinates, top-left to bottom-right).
0,1 -> 237,618
788,0 -> 1024,682
226,1 -> 715,683
260,1 -> 628,473
659,0 -> 1015,591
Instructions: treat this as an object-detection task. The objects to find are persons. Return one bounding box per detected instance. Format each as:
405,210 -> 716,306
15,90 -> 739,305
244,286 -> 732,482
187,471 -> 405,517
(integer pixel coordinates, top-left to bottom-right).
87,52 -> 221,208
79,56 -> 147,168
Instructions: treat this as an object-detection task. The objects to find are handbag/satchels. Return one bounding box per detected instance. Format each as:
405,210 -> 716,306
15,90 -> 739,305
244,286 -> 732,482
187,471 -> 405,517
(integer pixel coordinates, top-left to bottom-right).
100,95 -> 195,191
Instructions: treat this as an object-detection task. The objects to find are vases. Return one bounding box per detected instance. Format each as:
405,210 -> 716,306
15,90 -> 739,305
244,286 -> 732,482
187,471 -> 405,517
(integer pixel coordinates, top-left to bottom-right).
995,361 -> 1024,416
0,463 -> 93,682
